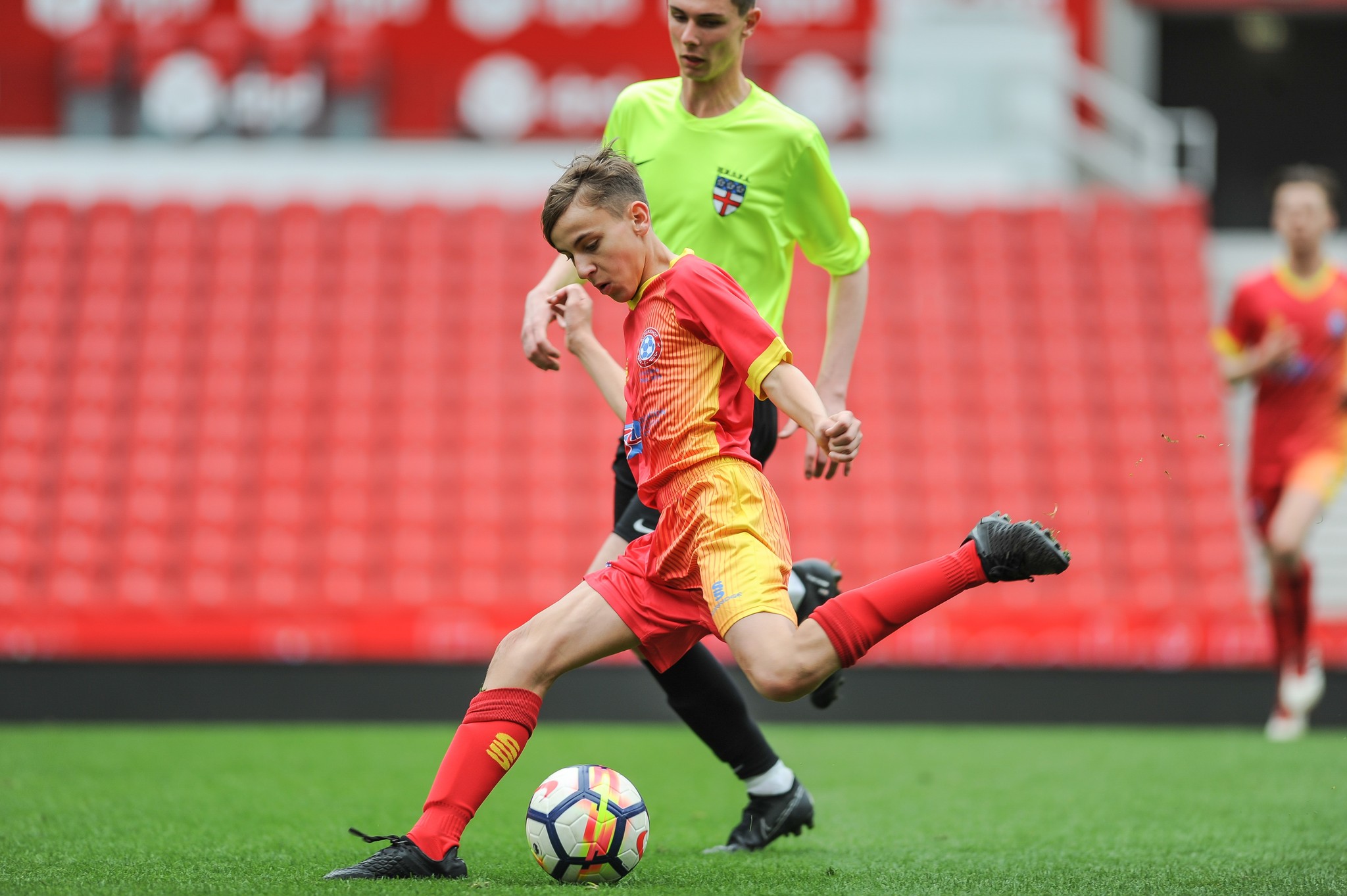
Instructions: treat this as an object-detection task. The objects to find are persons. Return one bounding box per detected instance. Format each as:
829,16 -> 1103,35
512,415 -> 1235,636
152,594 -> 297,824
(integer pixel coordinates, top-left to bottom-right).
1213,167 -> 1346,740
323,149 -> 1071,882
520,0 -> 870,853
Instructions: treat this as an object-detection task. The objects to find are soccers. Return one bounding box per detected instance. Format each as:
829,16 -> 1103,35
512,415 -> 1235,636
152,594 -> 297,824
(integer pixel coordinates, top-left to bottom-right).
524,763 -> 650,884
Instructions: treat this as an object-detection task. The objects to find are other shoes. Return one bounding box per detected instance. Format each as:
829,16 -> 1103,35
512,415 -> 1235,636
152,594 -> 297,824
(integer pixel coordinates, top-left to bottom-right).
1278,644 -> 1323,712
1267,703 -> 1306,744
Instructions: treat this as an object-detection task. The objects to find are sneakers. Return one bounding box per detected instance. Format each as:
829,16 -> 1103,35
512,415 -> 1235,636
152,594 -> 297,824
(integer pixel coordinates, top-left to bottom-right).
791,557 -> 846,708
956,510 -> 1070,584
322,827 -> 468,879
701,772 -> 814,853
